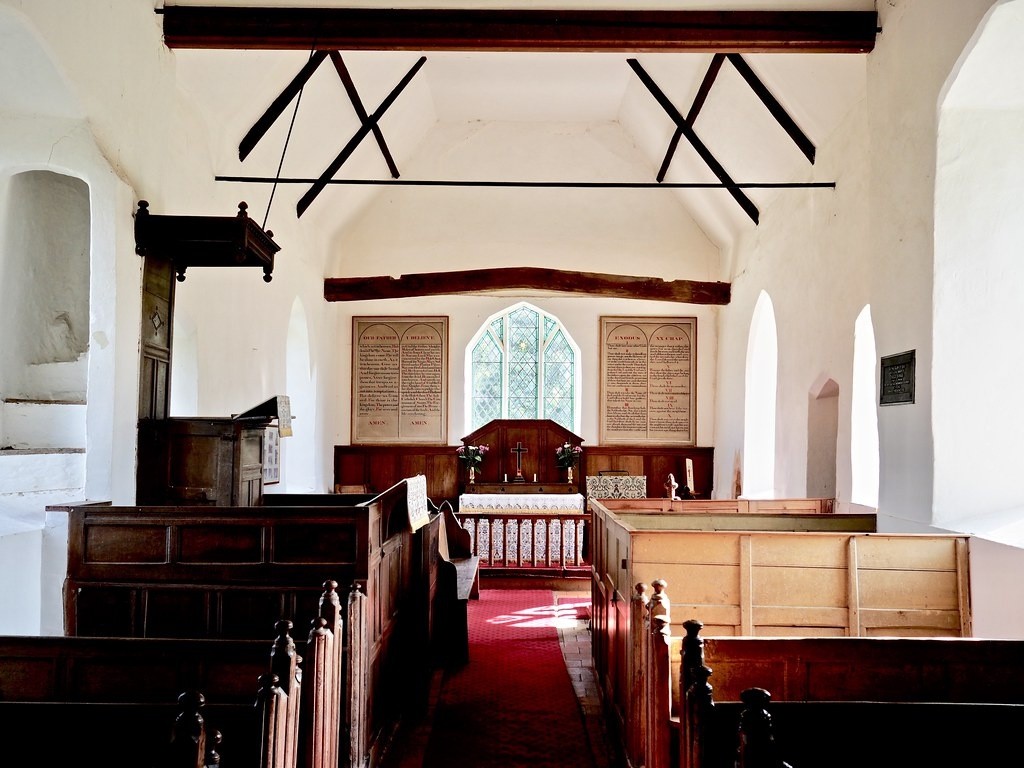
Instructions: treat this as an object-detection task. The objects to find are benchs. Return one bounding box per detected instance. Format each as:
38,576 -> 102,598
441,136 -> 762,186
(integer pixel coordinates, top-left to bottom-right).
667,637 -> 1024,768
0,581 -> 349,768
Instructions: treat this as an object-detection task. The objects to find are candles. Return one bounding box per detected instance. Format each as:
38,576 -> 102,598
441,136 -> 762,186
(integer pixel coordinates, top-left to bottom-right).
505,474 -> 507,481
534,474 -> 536,481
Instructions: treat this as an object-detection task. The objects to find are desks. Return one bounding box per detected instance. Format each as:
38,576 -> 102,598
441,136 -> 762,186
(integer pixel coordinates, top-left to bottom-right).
461,493 -> 585,562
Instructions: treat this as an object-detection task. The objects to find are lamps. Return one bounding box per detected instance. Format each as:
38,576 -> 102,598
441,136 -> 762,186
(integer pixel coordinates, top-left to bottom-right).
568,468 -> 573,483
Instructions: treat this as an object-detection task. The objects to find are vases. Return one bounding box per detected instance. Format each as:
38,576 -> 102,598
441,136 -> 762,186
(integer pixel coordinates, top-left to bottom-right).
470,467 -> 475,483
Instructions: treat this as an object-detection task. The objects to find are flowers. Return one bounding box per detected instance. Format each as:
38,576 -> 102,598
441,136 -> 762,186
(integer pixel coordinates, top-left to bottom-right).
556,442 -> 583,467
456,445 -> 489,474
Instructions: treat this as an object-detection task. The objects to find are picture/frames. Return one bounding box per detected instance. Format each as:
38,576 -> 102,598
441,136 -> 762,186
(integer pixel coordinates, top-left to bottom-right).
880,348 -> 916,406
